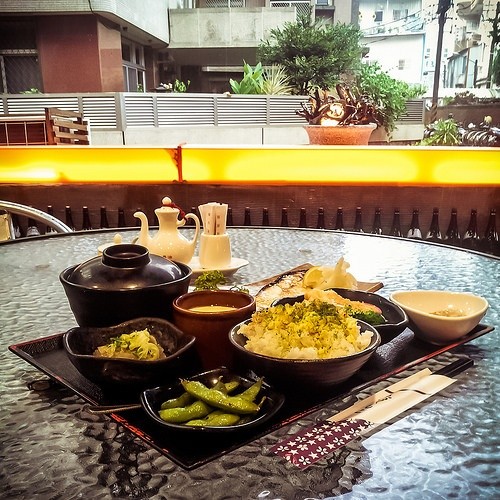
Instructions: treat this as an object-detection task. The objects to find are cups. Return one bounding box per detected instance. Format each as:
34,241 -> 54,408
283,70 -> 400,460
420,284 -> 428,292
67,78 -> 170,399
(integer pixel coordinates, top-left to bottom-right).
199,232 -> 232,269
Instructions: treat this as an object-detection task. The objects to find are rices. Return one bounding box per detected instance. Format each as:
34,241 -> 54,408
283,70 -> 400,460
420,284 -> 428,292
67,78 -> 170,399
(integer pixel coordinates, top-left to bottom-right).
237,321 -> 374,359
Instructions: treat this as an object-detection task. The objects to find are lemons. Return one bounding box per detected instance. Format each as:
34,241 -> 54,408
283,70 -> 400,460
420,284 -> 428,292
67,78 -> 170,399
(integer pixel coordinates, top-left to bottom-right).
304,266 -> 326,288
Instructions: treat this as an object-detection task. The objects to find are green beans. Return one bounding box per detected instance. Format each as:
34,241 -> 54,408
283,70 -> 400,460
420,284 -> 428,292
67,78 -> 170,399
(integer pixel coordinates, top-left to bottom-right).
158,376 -> 267,427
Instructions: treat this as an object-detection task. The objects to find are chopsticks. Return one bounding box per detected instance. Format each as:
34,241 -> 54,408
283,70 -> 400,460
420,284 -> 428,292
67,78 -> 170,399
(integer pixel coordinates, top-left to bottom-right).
434,357 -> 474,379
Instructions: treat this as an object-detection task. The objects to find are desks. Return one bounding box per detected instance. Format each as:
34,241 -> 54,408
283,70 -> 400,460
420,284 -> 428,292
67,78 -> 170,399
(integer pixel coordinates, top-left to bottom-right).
0,225 -> 500,500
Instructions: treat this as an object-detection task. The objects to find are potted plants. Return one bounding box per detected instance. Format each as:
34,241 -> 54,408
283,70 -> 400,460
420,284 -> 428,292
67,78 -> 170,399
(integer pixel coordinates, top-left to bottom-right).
295,84 -> 377,145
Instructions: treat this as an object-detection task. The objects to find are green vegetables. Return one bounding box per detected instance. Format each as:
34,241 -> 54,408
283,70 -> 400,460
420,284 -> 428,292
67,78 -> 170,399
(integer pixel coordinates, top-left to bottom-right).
277,299 -> 385,325
110,337 -> 126,356
193,268 -> 250,294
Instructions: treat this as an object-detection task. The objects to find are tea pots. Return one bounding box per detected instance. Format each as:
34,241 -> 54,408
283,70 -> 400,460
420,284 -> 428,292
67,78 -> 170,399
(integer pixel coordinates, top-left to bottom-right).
134,196 -> 200,267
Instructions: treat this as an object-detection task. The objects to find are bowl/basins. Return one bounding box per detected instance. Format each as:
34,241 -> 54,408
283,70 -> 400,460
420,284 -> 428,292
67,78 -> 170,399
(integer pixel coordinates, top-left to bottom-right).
271,286 -> 411,352
228,313 -> 381,387
172,288 -> 258,370
388,289 -> 489,347
140,368 -> 284,433
62,314 -> 198,392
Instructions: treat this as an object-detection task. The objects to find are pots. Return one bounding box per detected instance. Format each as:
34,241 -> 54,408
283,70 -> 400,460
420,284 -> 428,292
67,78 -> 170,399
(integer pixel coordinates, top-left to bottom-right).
59,244 -> 193,335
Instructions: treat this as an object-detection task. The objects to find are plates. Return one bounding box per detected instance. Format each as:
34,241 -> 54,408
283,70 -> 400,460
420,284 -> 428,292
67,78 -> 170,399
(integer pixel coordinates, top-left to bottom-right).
186,257 -> 250,279
237,262 -> 385,314
